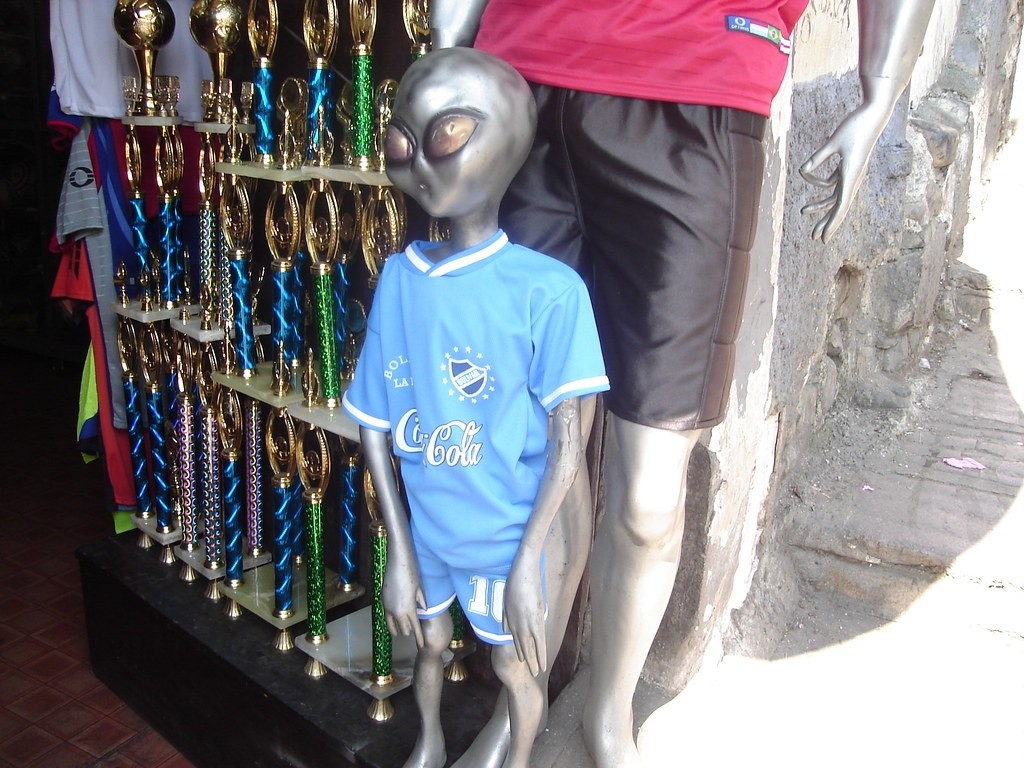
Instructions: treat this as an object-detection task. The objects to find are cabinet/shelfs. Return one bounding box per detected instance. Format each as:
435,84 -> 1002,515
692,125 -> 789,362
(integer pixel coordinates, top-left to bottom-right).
0,0 -> 91,372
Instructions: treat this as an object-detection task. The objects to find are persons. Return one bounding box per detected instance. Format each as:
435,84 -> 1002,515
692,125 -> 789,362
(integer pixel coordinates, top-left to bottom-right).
341,46 -> 610,768
436,0 -> 935,768
